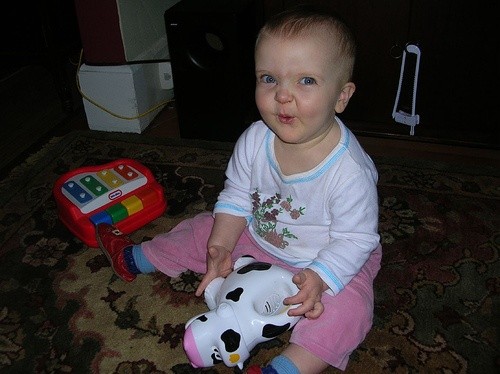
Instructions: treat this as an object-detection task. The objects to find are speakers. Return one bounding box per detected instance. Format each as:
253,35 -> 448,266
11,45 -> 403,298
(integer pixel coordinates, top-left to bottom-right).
164,0 -> 266,141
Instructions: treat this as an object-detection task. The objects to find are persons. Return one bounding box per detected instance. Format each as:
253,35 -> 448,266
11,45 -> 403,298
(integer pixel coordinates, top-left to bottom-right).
95,14 -> 382,374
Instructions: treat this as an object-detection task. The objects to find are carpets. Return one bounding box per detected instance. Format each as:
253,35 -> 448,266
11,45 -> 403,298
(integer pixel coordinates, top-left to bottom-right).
0,131 -> 500,374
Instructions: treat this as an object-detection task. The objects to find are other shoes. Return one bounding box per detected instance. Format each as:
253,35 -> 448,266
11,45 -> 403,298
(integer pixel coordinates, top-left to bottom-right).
96,222 -> 137,283
243,362 -> 262,374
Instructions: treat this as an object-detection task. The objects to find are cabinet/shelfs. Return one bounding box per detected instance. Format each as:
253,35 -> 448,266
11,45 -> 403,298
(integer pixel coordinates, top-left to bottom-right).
264,1 -> 500,175
